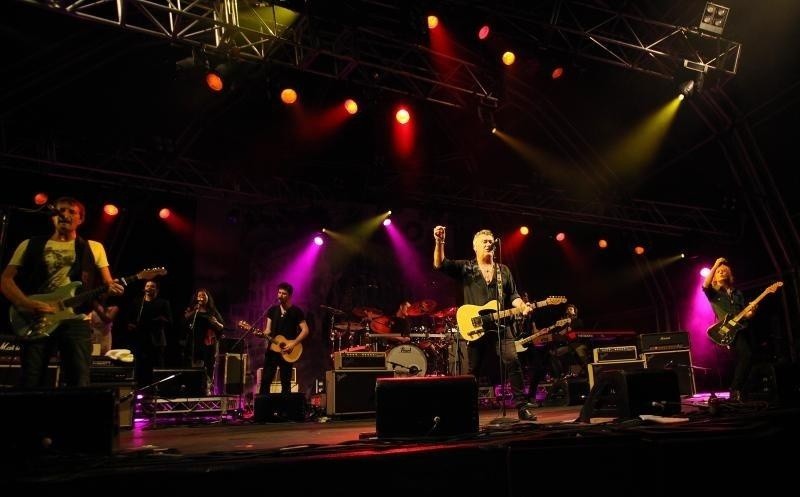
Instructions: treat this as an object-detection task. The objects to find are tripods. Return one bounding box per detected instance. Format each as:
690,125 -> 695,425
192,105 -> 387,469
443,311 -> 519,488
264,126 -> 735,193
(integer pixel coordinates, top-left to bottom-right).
213,333 -> 254,425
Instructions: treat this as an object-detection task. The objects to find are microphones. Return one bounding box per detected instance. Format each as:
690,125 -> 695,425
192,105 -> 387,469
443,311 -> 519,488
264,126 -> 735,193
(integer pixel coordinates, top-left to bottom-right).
320,304 -> 342,313
488,238 -> 498,251
196,297 -> 204,301
272,297 -> 284,304
677,363 -> 691,368
120,393 -> 134,402
179,385 -> 187,392
142,289 -> 151,294
49,205 -> 68,223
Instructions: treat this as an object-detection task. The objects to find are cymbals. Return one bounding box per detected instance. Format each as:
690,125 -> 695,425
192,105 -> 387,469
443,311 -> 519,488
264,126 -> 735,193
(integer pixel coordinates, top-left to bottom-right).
334,300 -> 456,332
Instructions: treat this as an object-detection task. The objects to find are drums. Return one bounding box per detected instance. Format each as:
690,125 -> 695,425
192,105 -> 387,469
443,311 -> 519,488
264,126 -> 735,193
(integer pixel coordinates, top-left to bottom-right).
385,342 -> 427,376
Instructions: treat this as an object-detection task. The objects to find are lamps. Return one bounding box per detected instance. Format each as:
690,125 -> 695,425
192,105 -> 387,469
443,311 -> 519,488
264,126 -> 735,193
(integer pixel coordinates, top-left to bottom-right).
700,0 -> 731,35
673,69 -> 706,100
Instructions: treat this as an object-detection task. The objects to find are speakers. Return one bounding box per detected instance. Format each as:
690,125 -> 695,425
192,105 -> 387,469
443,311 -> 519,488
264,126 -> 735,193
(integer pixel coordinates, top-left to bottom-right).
744,362 -> 800,408
325,369 -> 394,418
377,374 -> 479,441
89,382 -> 134,429
0,386 -> 120,456
254,393 -> 305,423
639,349 -> 696,395
587,360 -> 645,393
154,369 -> 207,396
579,368 -> 681,420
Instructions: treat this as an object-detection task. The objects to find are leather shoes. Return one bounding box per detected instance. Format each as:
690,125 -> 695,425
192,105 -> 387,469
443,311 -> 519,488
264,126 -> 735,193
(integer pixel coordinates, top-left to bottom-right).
517,409 -> 538,422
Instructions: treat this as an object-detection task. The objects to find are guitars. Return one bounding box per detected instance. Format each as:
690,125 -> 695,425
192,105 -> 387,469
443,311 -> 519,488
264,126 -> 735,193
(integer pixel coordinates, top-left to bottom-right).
238,320 -> 303,364
8,267 -> 169,340
497,319 -> 571,364
455,296 -> 568,342
705,282 -> 784,346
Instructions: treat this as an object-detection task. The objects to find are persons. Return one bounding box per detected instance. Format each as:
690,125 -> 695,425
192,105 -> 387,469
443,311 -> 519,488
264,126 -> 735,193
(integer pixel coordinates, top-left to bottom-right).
566,303 -> 583,331
434,224 -> 537,421
513,291 -> 539,335
702,257 -> 759,359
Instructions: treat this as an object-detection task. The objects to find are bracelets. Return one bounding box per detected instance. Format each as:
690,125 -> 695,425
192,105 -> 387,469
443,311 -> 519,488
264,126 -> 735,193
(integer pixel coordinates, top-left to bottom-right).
436,242 -> 445,245
